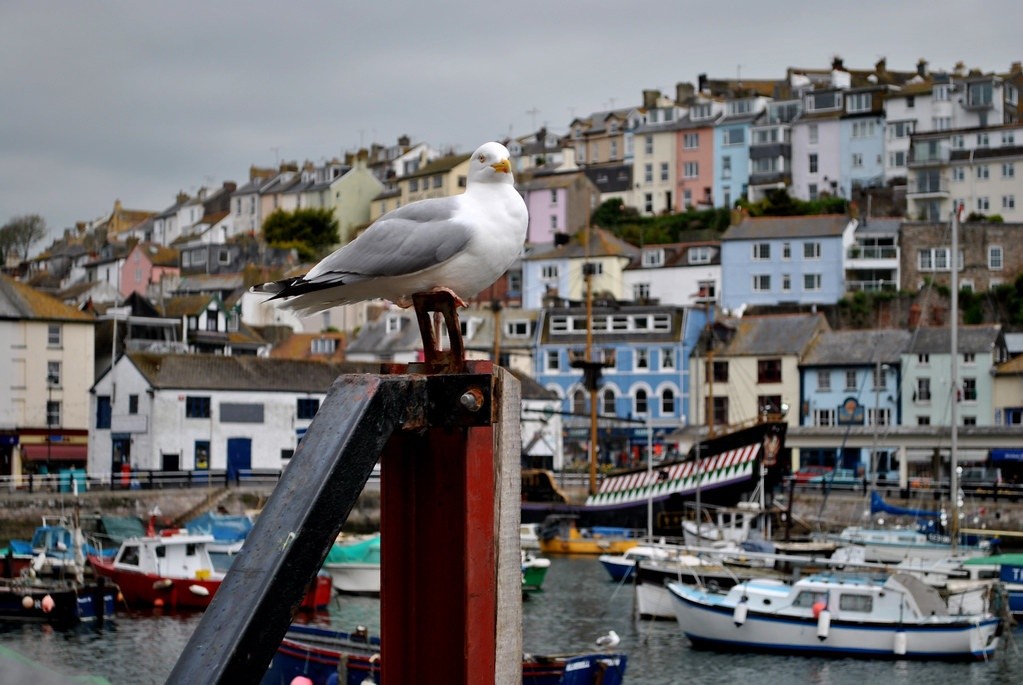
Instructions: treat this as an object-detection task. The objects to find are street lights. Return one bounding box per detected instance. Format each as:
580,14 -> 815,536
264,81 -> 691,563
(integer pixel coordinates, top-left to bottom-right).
907,189 -> 958,530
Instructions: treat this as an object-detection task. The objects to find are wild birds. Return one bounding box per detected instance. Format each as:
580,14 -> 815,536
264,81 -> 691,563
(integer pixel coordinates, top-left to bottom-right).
253,142 -> 532,319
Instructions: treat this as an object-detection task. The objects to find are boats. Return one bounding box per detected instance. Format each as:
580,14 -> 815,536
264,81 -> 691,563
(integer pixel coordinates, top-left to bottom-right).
0,210 -> 1022,657
271,621 -> 627,684
